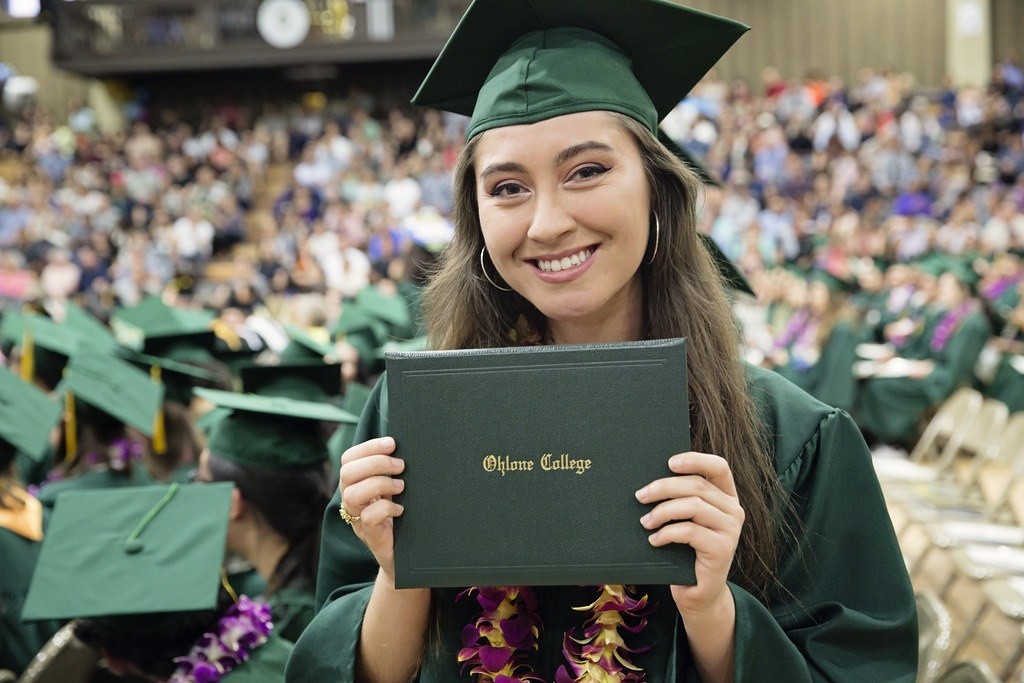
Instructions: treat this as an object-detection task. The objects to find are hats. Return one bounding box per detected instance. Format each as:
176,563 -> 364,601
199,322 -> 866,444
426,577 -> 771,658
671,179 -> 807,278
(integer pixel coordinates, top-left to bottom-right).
55,345 -> 164,439
121,346 -> 221,384
760,257 -> 846,296
65,302 -> 138,363
867,246 -> 987,288
0,365 -> 61,465
0,309 -> 69,372
111,293 -> 215,353
331,279 -> 431,376
190,386 -> 360,473
279,324 -> 329,366
326,383 -> 371,481
410,0 -> 752,146
20,482 -> 234,641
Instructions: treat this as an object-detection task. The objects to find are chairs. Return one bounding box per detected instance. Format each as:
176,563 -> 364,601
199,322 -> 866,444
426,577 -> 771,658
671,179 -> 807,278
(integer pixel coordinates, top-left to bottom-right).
934,545 -> 1024,600
915,587 -> 952,683
894,409 -> 1024,580
943,575 -> 1024,683
928,659 -> 1004,683
880,396 -> 1010,542
872,386 -> 984,502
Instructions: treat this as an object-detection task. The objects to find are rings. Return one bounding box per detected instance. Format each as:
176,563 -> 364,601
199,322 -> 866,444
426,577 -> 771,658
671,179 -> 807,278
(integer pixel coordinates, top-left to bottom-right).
339,499 -> 361,523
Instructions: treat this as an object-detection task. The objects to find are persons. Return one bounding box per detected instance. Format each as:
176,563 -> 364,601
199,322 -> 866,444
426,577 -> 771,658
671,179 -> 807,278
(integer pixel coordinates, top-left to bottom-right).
193,386 -> 362,644
1,0 -> 1023,683
288,0 -> 920,683
19,480 -> 296,682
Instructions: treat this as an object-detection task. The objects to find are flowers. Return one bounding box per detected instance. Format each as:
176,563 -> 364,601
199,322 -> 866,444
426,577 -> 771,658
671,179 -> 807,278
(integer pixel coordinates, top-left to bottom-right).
168,594 -> 274,683
458,583 -> 660,683
